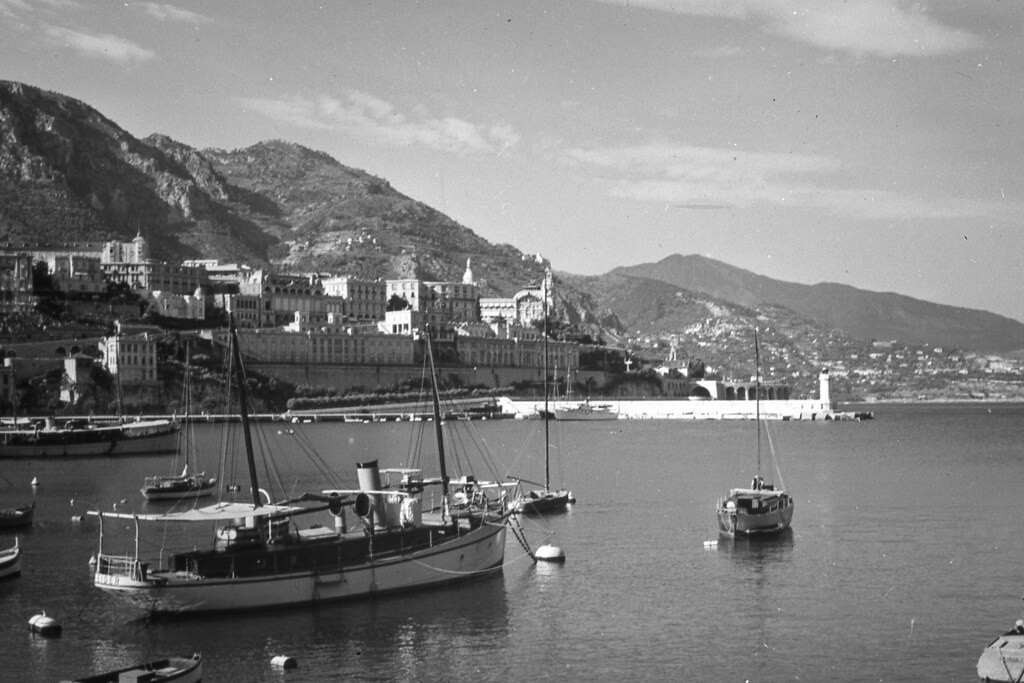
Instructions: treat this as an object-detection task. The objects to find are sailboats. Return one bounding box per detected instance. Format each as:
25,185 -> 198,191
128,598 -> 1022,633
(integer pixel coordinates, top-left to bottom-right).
715,332 -> 795,545
517,349 -> 620,422
86,302 -> 565,625
504,271 -> 577,516
0,325 -> 182,461
139,340 -> 221,503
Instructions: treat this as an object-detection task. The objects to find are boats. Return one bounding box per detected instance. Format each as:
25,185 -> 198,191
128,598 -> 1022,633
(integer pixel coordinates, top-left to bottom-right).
0,498 -> 36,531
978,616 -> 1024,683
56,650 -> 204,683
0,535 -> 23,581
26,609 -> 62,636
